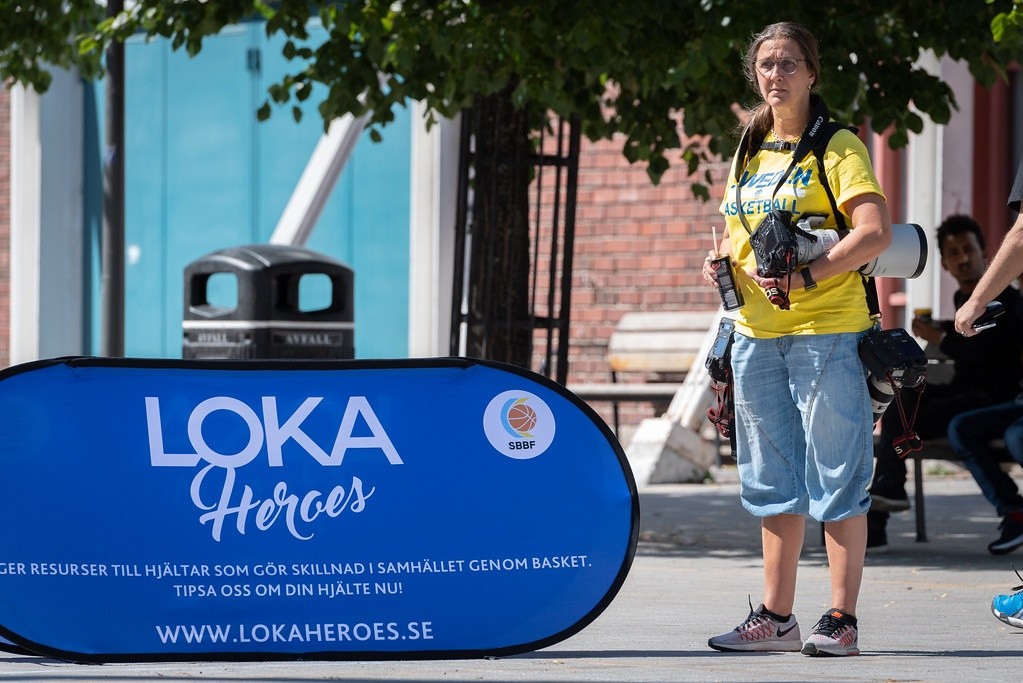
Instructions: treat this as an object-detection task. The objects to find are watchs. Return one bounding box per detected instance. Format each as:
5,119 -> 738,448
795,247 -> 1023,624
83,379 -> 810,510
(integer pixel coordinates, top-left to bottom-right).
800,265 -> 817,291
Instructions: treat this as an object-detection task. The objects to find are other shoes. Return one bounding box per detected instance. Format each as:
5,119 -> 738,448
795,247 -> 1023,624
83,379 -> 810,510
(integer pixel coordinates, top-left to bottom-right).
821,528 -> 889,555
865,481 -> 912,512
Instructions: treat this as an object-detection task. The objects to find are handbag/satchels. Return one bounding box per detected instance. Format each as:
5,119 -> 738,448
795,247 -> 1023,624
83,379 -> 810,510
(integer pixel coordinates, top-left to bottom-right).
705,316 -> 736,382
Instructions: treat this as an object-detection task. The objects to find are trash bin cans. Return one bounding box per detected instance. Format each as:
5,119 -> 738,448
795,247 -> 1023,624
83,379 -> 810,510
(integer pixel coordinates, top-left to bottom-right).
182,245 -> 355,358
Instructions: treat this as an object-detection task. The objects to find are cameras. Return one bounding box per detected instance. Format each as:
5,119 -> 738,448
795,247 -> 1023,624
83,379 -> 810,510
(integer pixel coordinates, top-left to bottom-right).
705,315 -> 738,462
749,210 -> 928,279
858,327 -> 929,432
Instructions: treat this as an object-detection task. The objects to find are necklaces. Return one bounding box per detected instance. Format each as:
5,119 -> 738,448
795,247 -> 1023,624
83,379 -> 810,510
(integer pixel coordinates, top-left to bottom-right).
770,123 -> 808,142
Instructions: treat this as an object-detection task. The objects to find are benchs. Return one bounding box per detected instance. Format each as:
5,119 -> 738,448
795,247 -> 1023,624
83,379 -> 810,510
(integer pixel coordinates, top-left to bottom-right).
566,308 -> 716,438
819,436 -> 1014,545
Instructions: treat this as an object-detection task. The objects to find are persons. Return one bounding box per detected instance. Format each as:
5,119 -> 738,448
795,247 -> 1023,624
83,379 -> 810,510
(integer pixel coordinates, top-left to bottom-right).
867,213 -> 1023,558
705,19 -> 890,658
954,164 -> 1023,628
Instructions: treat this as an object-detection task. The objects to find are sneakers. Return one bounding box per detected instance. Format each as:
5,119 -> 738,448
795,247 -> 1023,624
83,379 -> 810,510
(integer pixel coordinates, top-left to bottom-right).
801,609 -> 861,657
988,507 -> 1023,555
991,562 -> 1023,630
707,595 -> 803,653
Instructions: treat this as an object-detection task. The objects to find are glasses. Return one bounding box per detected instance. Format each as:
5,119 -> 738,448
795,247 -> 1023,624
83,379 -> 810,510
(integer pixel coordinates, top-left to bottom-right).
752,56 -> 812,75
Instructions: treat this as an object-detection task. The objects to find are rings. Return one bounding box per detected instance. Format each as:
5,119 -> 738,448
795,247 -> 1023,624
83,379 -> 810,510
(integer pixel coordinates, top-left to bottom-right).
961,331 -> 965,335
